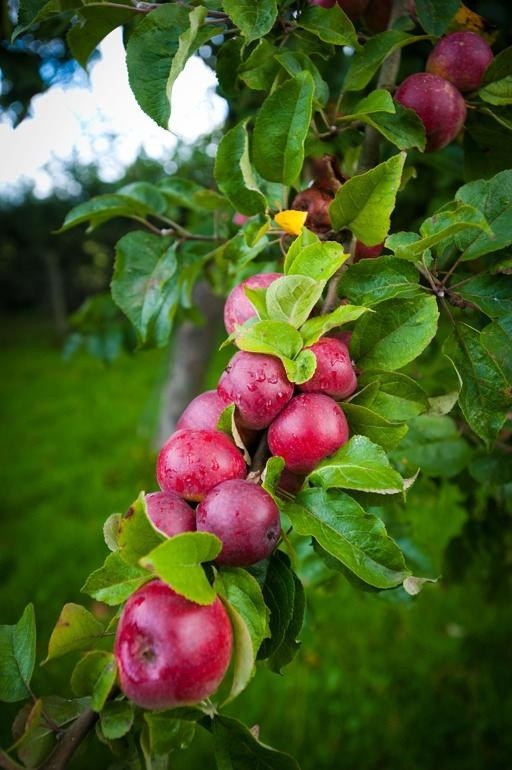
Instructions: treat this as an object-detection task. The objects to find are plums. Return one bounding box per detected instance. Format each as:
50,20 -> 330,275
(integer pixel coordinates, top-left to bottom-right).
288,1 -> 498,264
116,273 -> 363,711
